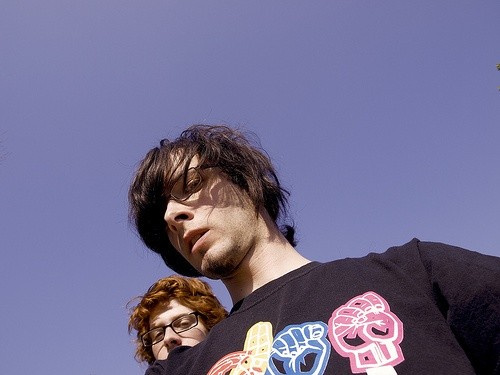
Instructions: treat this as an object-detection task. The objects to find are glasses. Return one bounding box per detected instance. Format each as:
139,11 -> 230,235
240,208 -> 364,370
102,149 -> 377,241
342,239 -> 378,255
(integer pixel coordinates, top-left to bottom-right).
148,162 -> 224,236
141,311 -> 199,348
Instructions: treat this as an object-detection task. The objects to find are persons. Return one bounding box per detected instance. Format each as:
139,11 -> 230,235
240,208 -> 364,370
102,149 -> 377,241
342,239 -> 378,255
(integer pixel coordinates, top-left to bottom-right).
126,124 -> 500,375
126,272 -> 229,365
